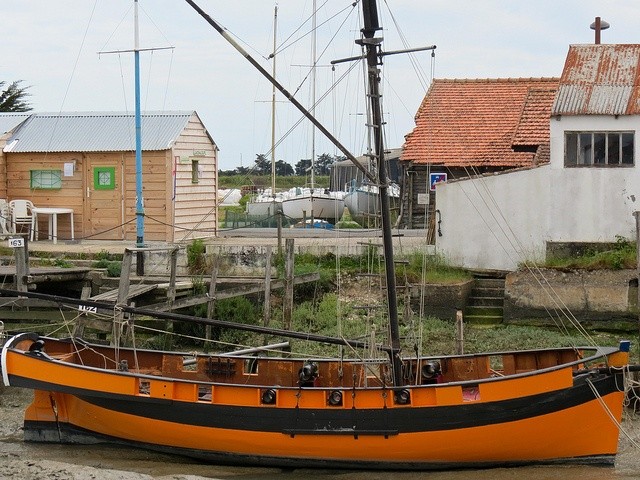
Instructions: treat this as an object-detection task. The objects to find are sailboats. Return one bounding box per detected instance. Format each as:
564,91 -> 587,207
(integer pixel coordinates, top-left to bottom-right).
344,0 -> 400,227
247,7 -> 284,222
282,1 -> 344,220
0,0 -> 629,471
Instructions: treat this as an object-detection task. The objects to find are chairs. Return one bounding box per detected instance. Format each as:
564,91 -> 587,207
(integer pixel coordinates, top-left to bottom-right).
7,199 -> 39,239
1,197 -> 9,236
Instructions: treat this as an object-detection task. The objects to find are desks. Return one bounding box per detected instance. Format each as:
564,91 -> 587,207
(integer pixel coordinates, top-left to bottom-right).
32,207 -> 75,244
